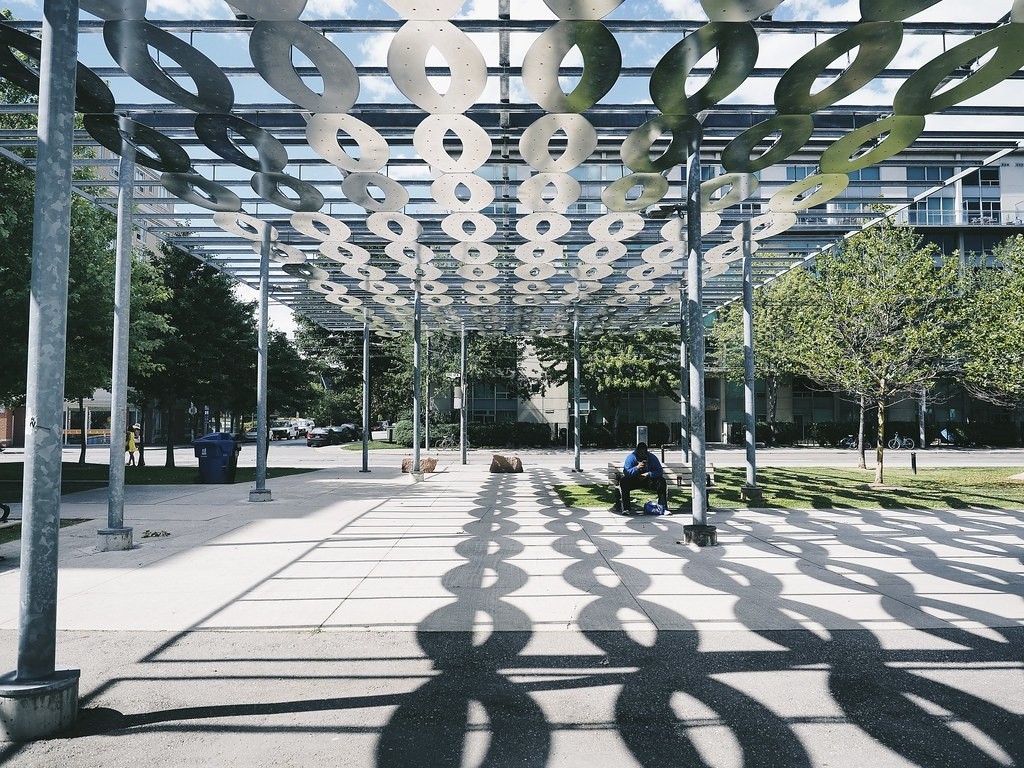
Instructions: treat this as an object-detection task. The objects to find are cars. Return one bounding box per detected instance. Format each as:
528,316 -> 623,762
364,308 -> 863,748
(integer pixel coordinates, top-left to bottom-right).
245,426 -> 273,441
371,420 -> 389,431
306,422 -> 362,447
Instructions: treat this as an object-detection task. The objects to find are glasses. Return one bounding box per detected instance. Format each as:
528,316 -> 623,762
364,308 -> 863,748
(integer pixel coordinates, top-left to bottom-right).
638,452 -> 647,455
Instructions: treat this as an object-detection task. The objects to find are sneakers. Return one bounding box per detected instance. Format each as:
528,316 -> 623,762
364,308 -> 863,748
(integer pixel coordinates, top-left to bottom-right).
664,509 -> 672,515
622,510 -> 632,515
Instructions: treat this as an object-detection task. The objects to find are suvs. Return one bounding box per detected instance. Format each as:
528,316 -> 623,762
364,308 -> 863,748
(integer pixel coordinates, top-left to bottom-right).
271,417 -> 315,440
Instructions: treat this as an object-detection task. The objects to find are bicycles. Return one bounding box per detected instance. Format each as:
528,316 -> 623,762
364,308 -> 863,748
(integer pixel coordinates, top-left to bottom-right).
888,431 -> 915,450
839,431 -> 872,450
435,433 -> 470,451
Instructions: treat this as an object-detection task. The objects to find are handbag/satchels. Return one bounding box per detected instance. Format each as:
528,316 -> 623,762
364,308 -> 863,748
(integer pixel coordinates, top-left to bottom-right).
125,445 -> 129,451
644,501 -> 664,515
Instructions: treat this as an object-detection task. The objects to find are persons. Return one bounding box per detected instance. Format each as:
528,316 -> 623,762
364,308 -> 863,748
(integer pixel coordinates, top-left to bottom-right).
619,442 -> 671,515
134,428 -> 146,466
126,426 -> 136,466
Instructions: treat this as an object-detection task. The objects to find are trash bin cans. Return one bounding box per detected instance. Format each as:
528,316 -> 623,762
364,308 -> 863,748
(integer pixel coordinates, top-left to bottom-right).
192,432 -> 239,486
